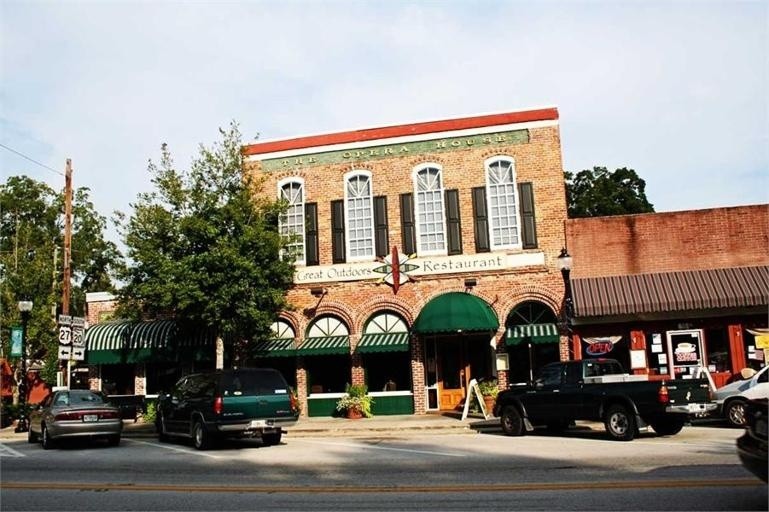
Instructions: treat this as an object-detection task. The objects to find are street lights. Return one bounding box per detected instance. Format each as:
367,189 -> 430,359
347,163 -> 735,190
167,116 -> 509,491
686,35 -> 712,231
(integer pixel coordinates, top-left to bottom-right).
556,246 -> 578,361
12,292 -> 34,435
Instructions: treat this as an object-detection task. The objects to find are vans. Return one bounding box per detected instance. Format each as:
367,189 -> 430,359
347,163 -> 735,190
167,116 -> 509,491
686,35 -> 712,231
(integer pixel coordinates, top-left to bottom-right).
150,364 -> 302,453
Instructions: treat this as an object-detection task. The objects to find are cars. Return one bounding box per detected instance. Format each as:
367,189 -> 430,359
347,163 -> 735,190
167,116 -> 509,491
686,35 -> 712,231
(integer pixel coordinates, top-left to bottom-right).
26,388 -> 126,451
708,363 -> 769,429
733,397 -> 768,485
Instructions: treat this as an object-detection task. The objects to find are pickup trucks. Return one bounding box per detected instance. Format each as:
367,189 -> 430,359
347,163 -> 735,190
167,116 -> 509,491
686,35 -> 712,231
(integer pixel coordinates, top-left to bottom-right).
492,355 -> 722,445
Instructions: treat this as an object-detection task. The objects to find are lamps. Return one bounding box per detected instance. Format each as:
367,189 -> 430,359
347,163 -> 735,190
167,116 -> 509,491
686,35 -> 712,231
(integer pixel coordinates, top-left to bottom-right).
311,287 -> 327,296
465,279 -> 476,285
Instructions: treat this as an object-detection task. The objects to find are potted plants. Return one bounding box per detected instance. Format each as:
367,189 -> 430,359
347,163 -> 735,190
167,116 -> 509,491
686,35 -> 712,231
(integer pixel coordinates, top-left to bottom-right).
336,382 -> 376,419
465,379 -> 501,416
